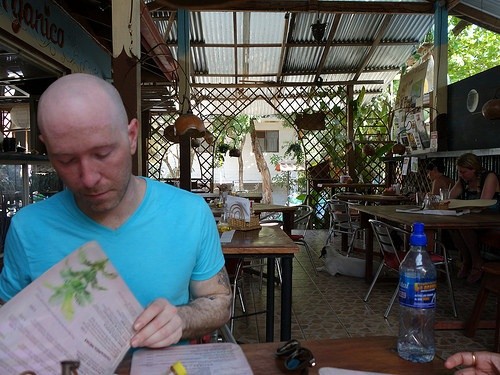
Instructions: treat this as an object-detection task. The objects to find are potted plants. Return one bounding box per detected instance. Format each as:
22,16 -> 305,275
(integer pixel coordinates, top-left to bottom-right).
212,115 -> 255,168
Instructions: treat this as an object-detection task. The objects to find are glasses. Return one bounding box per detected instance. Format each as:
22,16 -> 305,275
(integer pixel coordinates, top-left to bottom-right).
275,338 -> 317,375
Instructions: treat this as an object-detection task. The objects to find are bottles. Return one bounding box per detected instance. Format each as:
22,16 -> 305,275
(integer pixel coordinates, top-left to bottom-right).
397,223 -> 436,364
396,182 -> 400,194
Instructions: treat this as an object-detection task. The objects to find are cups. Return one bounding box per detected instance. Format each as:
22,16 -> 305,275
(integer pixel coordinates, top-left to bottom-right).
416,192 -> 426,208
439,188 -> 450,200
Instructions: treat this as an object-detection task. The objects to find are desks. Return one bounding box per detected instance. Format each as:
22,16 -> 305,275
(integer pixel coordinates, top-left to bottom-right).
317,182 -> 387,196
350,205 -> 500,330
113,336 -> 465,375
210,204 -> 299,236
341,193 -> 409,248
218,226 -> 300,342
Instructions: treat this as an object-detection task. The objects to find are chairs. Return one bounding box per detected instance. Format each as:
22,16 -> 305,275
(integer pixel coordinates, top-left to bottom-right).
225,220 -> 284,334
258,205 -> 320,277
323,192 -> 458,320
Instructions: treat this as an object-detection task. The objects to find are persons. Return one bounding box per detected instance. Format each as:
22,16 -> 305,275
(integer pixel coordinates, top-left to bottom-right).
408,158 -> 462,201
0,73 -> 232,350
448,153 -> 500,283
444,351 -> 500,375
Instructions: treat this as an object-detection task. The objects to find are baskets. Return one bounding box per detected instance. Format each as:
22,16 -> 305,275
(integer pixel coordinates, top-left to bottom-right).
227,203 -> 261,230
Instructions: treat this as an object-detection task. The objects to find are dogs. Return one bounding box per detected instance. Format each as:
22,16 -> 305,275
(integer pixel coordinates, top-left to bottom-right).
315,244 -> 385,279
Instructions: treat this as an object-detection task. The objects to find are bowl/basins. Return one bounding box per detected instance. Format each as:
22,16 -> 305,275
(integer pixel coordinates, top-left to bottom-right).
340,176 -> 351,184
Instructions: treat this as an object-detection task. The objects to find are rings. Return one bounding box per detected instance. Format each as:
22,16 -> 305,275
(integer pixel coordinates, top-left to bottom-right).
472,352 -> 476,366
